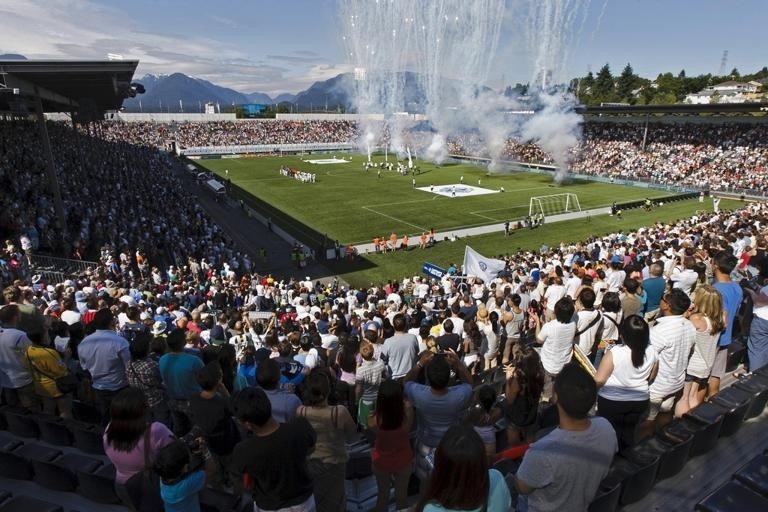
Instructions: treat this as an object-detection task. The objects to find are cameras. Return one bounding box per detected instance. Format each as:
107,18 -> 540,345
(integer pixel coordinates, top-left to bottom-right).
181,424 -> 204,451
433,353 -> 448,359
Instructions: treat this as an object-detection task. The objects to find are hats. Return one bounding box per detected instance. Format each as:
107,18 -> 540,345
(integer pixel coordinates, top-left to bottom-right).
305,276 -> 311,281
149,320 -> 168,335
156,306 -> 167,314
476,308 -> 489,321
254,348 -> 273,361
177,316 -> 189,328
75,291 -> 88,302
66,286 -> 75,296
109,288 -> 121,299
31,274 -> 42,284
210,325 -> 225,346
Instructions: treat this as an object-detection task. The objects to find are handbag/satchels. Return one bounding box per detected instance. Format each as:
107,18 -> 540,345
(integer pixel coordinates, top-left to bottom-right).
55,373 -> 82,394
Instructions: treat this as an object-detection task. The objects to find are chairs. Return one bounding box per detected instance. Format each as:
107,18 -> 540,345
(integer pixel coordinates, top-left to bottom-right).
1,401 -> 122,510
590,361 -> 767,511
475,366 -> 505,397
346,451 -> 373,479
493,415 -> 520,511
0,117 -> 228,278
535,400 -> 558,434
